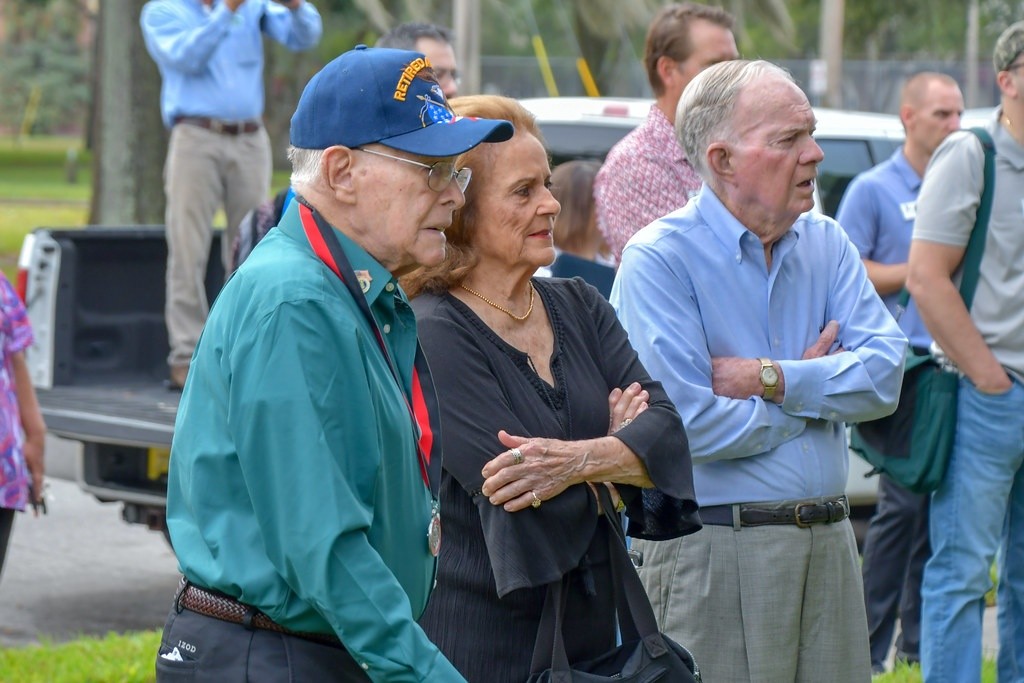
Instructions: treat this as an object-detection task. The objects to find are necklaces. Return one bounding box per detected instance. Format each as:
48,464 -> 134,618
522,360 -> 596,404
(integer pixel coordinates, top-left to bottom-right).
1004,116 -> 1012,126
458,281 -> 534,320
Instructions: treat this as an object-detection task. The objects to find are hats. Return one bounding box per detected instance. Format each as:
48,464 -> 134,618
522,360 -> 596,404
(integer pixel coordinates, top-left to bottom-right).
288,44 -> 515,156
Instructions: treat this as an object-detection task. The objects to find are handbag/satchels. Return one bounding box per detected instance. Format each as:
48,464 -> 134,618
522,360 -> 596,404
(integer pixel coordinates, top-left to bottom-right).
848,347 -> 958,493
529,481 -> 704,683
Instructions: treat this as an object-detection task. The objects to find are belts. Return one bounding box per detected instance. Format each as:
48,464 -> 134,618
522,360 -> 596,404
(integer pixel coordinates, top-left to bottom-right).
174,574 -> 344,646
699,495 -> 849,527
175,116 -> 261,134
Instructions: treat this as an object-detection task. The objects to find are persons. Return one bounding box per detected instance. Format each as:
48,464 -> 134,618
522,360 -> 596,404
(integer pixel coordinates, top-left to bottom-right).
410,94 -> 703,683
592,3 -> 741,257
836,72 -> 965,680
141,0 -> 323,384
0,273 -> 46,566
909,20 -> 1024,683
551,160 -> 618,270
374,21 -> 457,99
610,61 -> 909,683
154,42 -> 512,682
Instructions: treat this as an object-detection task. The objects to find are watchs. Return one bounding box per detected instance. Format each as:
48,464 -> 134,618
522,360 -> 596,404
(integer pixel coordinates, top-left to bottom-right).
758,358 -> 778,401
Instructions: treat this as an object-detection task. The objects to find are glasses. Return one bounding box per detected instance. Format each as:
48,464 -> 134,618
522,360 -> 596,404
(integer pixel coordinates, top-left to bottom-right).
351,147 -> 472,193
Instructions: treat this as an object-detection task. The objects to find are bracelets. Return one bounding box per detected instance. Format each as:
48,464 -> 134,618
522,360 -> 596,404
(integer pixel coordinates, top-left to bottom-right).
618,496 -> 624,512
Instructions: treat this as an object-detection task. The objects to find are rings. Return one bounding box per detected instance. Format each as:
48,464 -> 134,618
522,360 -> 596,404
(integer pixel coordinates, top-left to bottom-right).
623,419 -> 631,425
511,449 -> 523,463
532,492 -> 541,508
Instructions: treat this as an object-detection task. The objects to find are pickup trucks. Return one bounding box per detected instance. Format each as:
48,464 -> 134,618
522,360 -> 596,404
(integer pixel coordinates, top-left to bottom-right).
12,92 -> 908,535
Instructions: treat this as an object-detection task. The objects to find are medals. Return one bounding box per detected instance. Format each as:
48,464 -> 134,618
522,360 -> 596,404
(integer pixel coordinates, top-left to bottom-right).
428,515 -> 441,557
